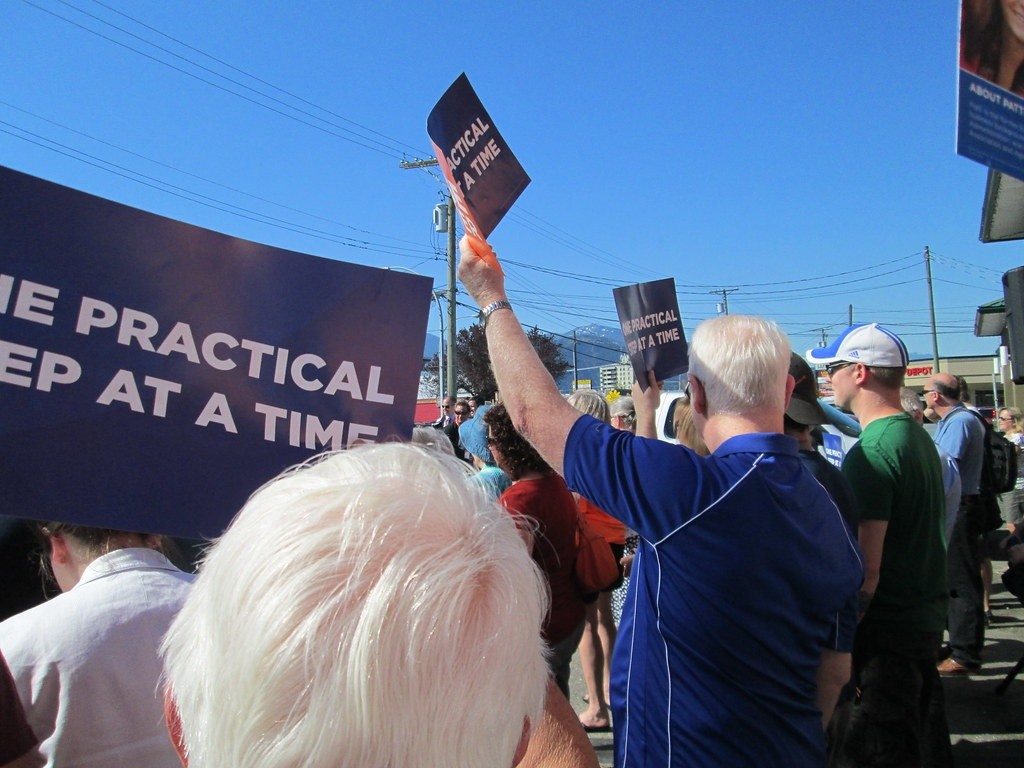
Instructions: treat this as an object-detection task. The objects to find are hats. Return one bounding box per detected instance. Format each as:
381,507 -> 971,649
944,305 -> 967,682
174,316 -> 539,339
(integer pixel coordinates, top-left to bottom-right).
458,404 -> 496,463
782,351 -> 827,427
806,322 -> 911,367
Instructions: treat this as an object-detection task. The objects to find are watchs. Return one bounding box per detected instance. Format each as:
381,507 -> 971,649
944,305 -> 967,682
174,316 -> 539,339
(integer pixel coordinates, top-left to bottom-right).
477,300 -> 513,337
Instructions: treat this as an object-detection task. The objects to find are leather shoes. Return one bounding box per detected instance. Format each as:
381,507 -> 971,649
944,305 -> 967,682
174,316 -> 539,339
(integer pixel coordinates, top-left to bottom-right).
938,657 -> 980,675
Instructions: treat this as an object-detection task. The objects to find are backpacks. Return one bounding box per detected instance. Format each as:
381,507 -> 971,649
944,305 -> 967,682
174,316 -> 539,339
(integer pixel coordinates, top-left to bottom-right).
967,409 -> 1018,493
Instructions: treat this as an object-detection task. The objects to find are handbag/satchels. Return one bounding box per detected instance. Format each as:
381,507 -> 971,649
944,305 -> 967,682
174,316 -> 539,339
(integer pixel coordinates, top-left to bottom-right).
575,520 -> 620,593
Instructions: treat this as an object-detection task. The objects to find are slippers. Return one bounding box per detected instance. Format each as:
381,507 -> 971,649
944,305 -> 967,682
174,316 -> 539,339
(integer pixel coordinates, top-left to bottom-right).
583,697 -> 611,710
582,724 -> 611,732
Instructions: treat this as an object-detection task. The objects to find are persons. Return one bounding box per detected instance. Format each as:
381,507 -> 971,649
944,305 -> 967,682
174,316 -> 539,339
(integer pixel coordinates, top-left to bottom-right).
160,441 -> 598,767
807,322 -> 951,768
0,372 -> 1022,768
960,0 -> 1023,98
456,233 -> 865,768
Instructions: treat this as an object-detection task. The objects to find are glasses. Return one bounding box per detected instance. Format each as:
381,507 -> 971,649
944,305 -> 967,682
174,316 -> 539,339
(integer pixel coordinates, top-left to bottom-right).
455,410 -> 469,416
442,405 -> 454,409
486,435 -> 497,445
999,417 -> 1013,421
684,381 -> 691,399
824,362 -> 857,374
922,390 -> 941,394
